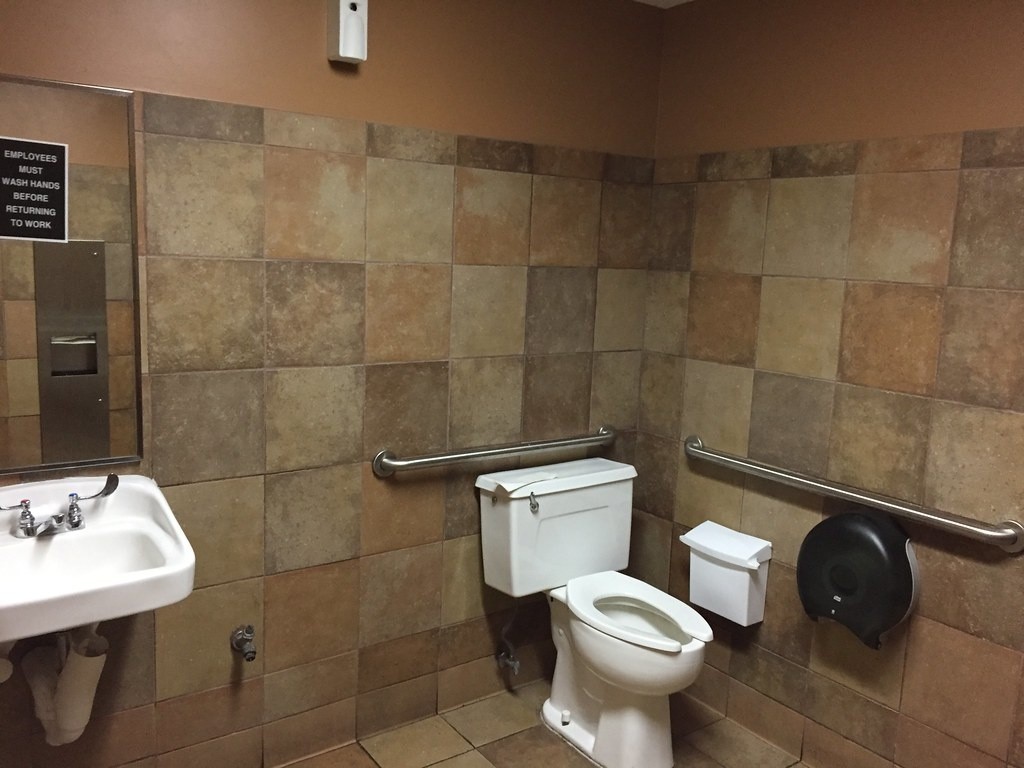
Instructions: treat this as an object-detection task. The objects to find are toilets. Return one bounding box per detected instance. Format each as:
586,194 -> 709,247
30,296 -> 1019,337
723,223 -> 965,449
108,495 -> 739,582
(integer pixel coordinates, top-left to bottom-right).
476,451 -> 715,768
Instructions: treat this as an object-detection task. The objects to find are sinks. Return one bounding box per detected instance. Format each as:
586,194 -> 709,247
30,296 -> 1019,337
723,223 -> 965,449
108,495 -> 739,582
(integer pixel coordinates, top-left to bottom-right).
0,472 -> 194,644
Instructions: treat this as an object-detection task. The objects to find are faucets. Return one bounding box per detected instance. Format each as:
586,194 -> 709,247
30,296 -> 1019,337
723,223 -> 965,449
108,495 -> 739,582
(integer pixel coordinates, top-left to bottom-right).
38,513 -> 67,536
